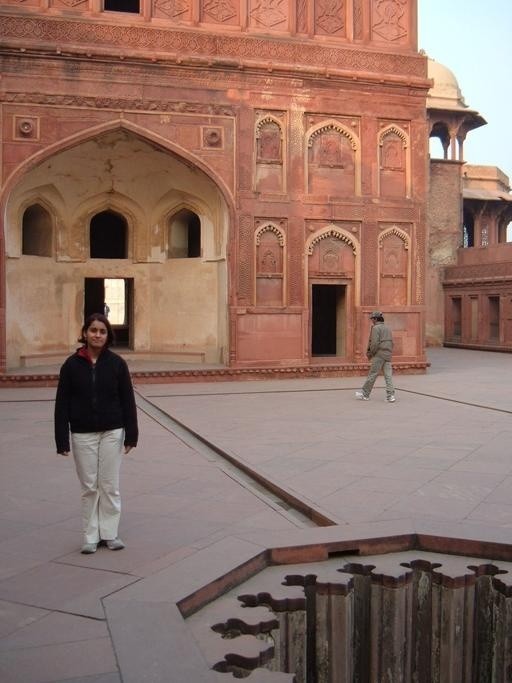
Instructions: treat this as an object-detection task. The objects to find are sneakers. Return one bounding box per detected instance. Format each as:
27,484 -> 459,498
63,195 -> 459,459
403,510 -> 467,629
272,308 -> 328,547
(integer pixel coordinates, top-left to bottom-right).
106,537 -> 125,550
81,543 -> 101,554
355,392 -> 369,401
384,396 -> 395,401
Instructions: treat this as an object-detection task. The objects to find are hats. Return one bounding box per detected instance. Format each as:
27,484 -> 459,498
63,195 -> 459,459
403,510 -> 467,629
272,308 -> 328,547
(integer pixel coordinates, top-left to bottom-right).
368,311 -> 383,320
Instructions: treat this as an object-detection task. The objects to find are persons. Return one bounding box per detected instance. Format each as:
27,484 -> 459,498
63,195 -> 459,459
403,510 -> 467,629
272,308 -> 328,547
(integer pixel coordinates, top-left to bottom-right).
104,303 -> 109,317
355,311 -> 396,402
54,313 -> 138,555
